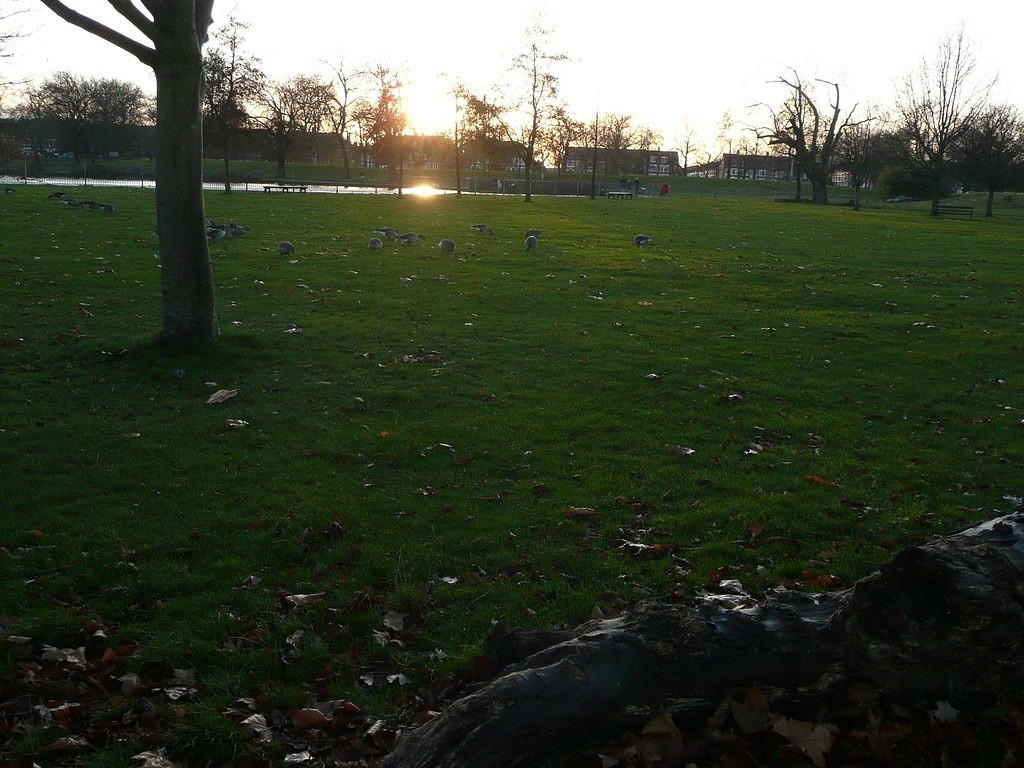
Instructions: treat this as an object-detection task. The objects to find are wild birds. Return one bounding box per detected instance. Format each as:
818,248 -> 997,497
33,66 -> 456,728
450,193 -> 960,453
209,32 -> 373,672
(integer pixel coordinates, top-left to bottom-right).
472,224 -> 494,235
367,226 -> 426,256
5,187 -> 16,194
204,220 -> 250,240
278,241 -> 295,257
632,235 -> 653,246
524,230 -> 542,252
48,192 -> 115,213
437,239 -> 455,254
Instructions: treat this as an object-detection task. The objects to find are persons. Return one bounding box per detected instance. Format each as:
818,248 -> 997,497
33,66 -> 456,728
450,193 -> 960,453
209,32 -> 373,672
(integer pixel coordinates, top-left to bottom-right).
659,183 -> 670,196
621,175 -> 639,188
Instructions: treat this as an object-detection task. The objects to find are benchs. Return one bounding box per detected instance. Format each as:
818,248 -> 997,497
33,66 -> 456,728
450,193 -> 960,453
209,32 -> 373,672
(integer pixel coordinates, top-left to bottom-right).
263,186 -> 308,193
933,205 -> 974,220
608,192 -> 633,200
